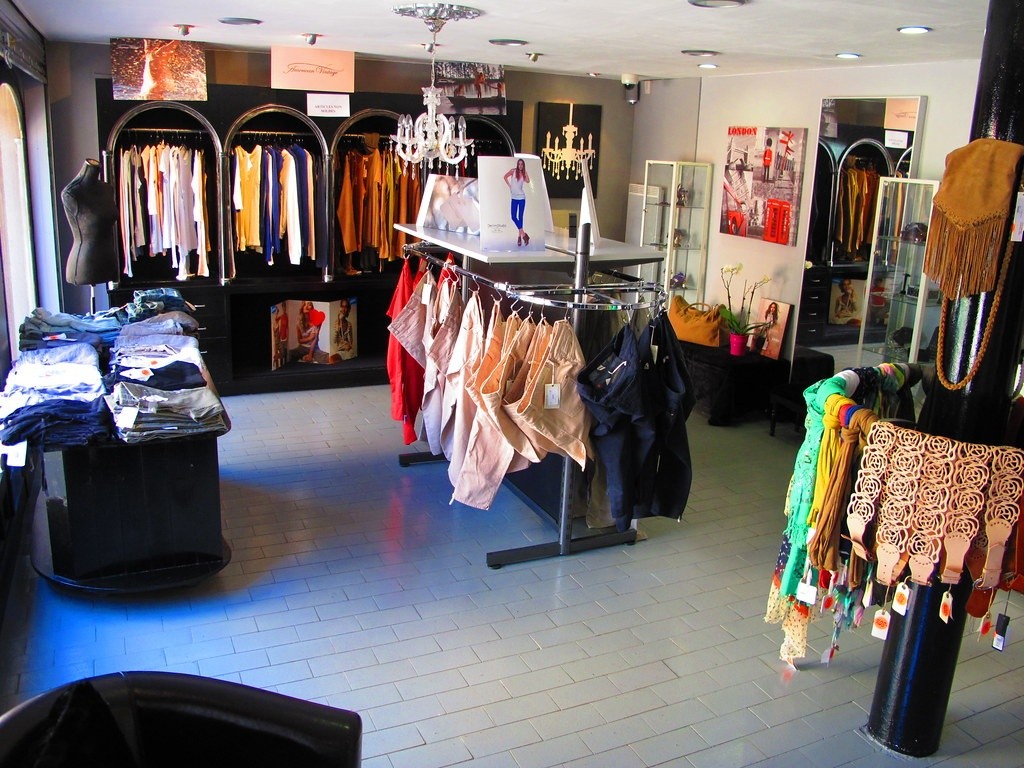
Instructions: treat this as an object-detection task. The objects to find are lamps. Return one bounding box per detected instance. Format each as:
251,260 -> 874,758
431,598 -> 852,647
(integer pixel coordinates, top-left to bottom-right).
390,4 -> 487,181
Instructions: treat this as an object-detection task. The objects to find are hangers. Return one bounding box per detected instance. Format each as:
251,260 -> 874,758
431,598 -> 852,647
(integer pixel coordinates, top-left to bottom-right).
400,245 -> 666,329
120,130 -> 502,158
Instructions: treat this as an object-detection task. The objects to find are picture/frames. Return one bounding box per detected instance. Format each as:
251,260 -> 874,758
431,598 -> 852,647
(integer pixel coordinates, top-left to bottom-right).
536,102 -> 601,199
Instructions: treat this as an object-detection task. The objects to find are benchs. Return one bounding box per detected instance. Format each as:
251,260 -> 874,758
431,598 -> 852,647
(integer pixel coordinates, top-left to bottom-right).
794,344 -> 834,426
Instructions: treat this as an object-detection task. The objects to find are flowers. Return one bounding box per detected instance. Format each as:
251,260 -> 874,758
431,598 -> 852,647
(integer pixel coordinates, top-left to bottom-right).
720,265 -> 770,324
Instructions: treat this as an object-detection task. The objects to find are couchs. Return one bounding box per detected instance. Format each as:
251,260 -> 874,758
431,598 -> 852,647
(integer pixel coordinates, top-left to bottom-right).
0,670 -> 362,767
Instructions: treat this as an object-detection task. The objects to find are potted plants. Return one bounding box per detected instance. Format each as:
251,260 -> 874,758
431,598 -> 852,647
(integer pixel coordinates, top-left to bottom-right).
720,309 -> 763,355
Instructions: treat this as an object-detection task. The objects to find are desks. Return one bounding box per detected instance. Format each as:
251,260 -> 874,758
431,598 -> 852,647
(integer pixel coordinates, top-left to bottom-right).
30,348 -> 230,595
678,339 -> 790,425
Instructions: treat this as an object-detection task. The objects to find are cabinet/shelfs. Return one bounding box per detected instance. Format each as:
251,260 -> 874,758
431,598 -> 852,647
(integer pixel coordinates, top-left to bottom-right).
637,160 -> 713,319
855,177 -> 942,370
796,121 -> 915,345
391,223 -> 665,567
96,75 -> 521,396
